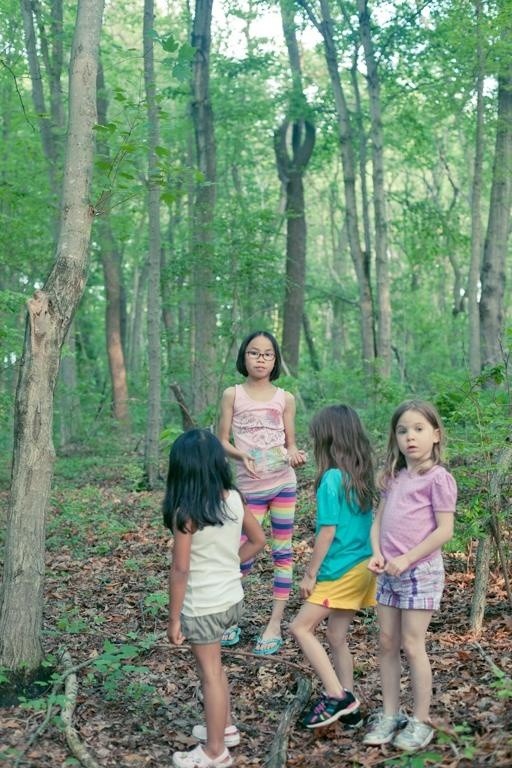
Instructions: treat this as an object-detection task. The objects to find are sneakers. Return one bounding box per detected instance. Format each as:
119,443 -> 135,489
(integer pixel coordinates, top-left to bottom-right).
302,686 -> 361,728
391,716 -> 438,754
361,711 -> 405,747
337,706 -> 364,730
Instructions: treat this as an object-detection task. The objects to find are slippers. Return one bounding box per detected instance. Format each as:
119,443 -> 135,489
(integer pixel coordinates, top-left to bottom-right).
251,636 -> 284,656
220,624 -> 243,647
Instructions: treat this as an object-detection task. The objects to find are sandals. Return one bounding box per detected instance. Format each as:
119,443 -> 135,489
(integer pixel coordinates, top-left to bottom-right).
171,743 -> 234,768
191,723 -> 241,748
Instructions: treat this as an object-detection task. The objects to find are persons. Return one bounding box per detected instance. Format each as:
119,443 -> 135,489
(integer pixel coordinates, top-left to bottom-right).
217,328 -> 312,656
361,397 -> 461,752
159,428 -> 269,767
285,402 -> 384,730
